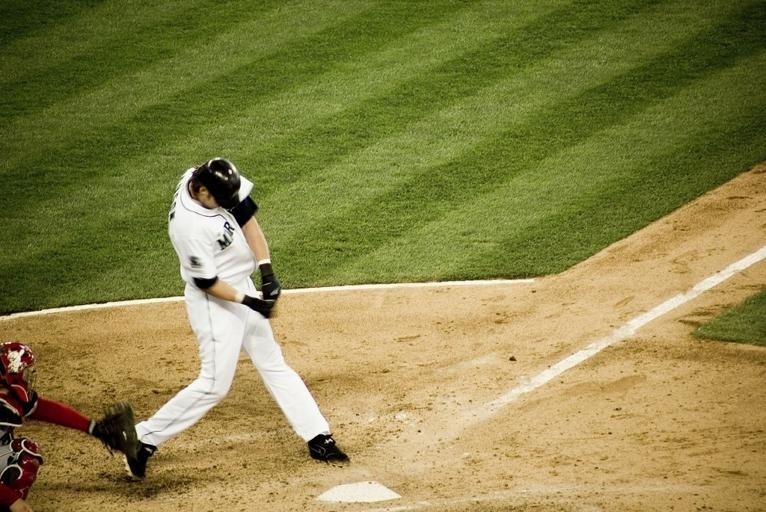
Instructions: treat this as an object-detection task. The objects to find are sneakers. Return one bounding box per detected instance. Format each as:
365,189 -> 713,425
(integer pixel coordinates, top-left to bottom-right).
308,433 -> 349,463
124,441 -> 157,479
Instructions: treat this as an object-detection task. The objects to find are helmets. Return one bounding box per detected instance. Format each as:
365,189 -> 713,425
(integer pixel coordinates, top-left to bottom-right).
202,158 -> 240,210
0,342 -> 40,395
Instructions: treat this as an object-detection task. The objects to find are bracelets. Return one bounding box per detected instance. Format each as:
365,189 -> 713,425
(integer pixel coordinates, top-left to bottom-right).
234,292 -> 246,303
258,258 -> 271,265
88,417 -> 96,433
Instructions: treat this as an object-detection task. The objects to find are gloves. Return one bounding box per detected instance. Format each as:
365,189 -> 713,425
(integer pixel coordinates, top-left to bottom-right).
258,262 -> 281,302
240,295 -> 273,318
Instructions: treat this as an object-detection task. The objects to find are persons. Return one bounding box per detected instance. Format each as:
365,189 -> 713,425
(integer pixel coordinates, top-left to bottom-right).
0,339 -> 139,512
114,156 -> 351,479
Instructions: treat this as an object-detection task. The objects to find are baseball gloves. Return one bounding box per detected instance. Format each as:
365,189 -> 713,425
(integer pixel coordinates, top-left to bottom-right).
102,403 -> 136,458
